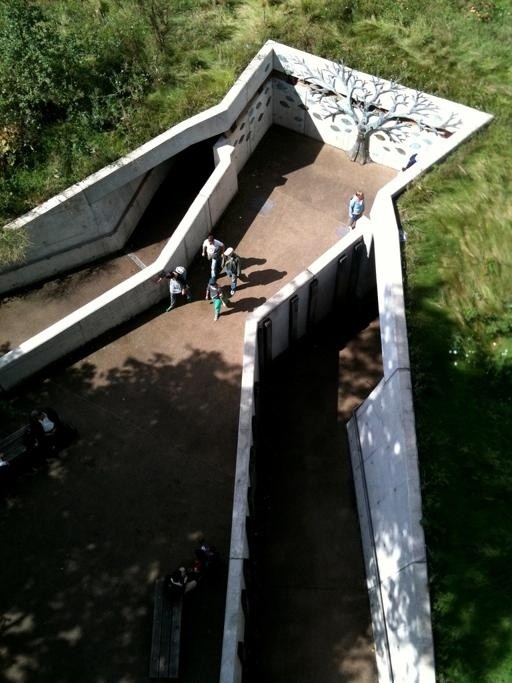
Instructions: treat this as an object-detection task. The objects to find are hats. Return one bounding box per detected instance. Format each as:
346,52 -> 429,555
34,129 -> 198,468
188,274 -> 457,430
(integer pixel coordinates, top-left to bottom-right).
209,278 -> 215,284
224,247 -> 233,256
356,192 -> 363,200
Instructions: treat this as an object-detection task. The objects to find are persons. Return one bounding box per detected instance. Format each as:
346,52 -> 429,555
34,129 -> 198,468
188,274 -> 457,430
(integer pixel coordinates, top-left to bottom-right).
164,537 -> 221,600
204,276 -> 230,321
201,232 -> 226,278
154,266 -> 194,313
346,190 -> 365,230
219,246 -> 243,296
1,410 -> 79,492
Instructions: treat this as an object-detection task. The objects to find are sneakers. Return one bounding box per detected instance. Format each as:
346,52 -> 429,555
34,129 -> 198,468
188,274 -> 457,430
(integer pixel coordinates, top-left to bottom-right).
231,291 -> 234,295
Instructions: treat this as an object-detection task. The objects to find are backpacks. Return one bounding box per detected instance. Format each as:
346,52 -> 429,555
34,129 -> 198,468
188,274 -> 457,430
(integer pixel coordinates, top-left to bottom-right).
175,267 -> 186,278
